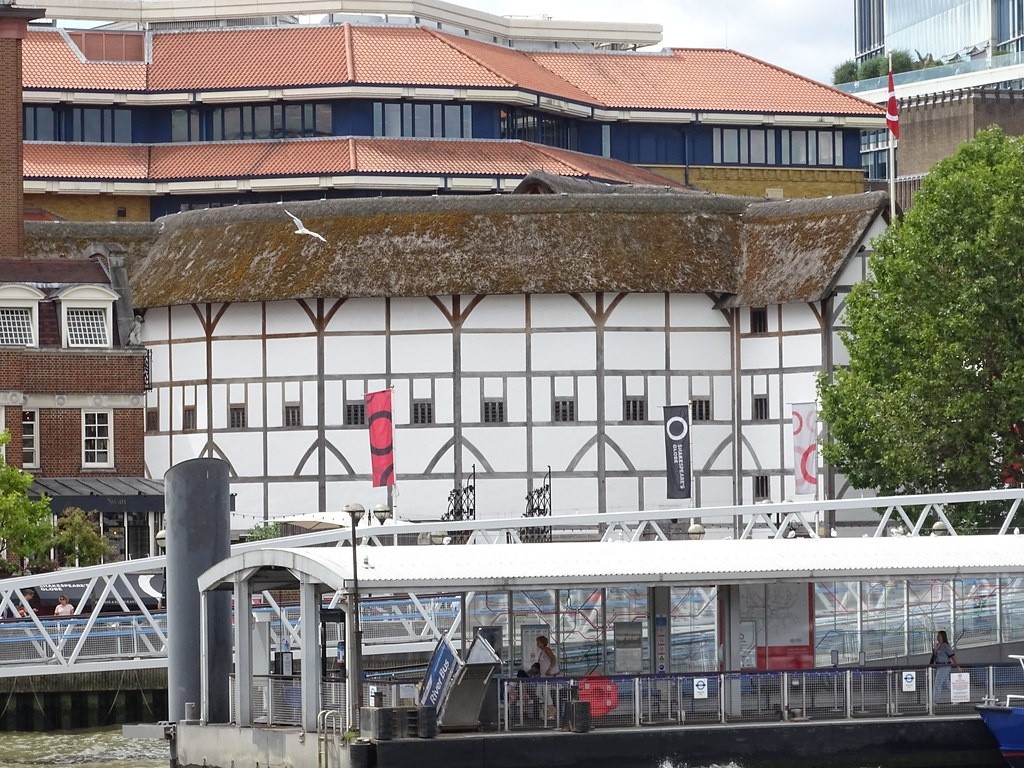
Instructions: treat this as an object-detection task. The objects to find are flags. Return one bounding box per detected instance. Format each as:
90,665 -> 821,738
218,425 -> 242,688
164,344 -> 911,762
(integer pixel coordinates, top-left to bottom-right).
792,403 -> 817,495
662,405 -> 691,499
886,72 -> 899,139
364,390 -> 394,487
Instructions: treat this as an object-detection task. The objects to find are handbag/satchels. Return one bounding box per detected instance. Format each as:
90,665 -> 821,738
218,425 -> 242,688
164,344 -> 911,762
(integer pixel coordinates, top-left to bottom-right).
544,655 -> 560,675
929,647 -> 937,669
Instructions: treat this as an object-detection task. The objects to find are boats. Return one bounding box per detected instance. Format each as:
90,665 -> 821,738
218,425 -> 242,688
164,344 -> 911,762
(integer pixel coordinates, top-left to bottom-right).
974,651 -> 1024,767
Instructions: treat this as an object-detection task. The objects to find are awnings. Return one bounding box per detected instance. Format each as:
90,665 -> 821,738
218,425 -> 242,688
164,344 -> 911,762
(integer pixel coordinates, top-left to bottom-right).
27,476 -> 234,514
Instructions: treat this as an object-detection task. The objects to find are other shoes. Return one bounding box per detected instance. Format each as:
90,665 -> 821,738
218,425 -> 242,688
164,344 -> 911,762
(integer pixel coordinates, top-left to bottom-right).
542,714 -> 555,721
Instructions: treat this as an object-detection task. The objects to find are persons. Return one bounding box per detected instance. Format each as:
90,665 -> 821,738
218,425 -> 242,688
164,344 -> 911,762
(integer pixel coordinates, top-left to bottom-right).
536,636 -> 559,720
933,631 -> 961,706
528,663 -> 540,719
19,589 -> 38,618
55,594 -> 74,615
